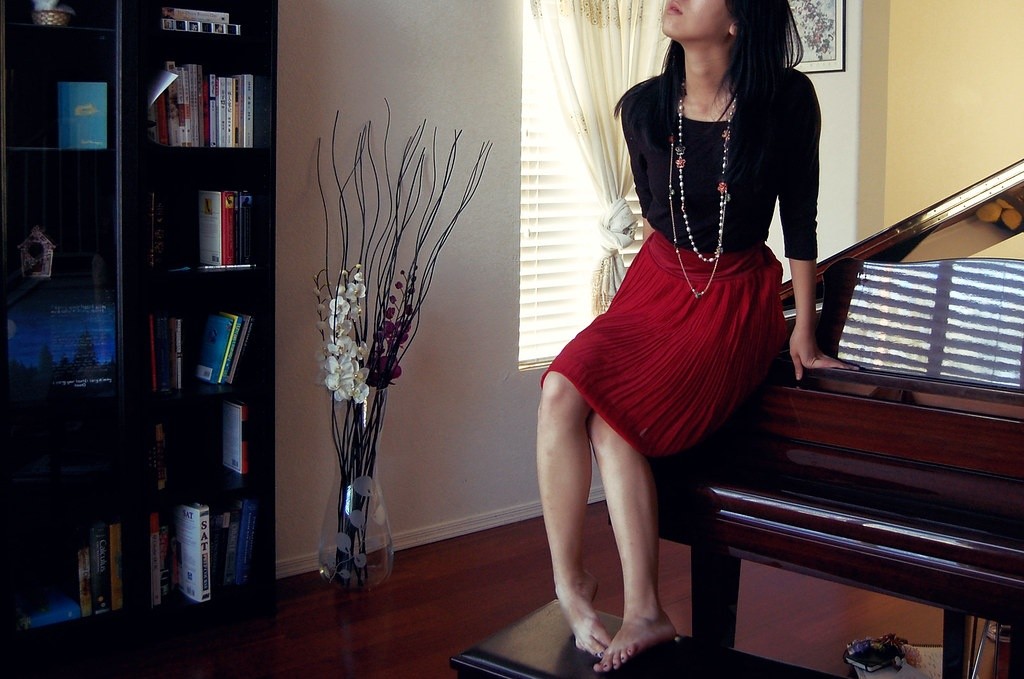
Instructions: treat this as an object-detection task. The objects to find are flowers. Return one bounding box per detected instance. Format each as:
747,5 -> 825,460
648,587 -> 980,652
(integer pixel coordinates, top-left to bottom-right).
314,97 -> 493,594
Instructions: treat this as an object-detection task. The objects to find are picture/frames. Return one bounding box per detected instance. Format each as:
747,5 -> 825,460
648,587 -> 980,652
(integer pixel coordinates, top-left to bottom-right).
785,0 -> 846,75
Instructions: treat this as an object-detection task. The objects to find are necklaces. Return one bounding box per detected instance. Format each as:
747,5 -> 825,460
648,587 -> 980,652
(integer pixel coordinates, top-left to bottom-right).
659,65 -> 741,300
674,56 -> 738,262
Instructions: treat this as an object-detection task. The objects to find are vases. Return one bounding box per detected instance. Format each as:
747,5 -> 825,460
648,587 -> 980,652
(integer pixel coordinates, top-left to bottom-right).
318,386 -> 396,593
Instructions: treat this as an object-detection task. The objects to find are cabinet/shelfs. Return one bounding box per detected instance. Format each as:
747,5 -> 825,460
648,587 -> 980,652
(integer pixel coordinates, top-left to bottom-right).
606,152 -> 1024,679
1,0 -> 279,651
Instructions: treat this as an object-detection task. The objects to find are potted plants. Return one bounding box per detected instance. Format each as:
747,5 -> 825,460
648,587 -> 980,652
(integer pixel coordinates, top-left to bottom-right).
845,634 -> 923,678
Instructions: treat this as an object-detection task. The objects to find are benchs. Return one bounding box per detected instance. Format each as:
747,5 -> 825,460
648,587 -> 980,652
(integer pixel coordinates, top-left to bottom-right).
449,598 -> 844,679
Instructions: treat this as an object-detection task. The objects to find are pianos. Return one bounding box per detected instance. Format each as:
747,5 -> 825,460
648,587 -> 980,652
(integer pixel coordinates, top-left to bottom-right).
649,156 -> 1024,679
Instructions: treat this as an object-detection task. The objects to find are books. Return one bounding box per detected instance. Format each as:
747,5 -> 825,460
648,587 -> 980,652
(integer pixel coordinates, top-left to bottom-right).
2,4 -> 281,629
853,639 -> 980,679
844,639 -> 905,673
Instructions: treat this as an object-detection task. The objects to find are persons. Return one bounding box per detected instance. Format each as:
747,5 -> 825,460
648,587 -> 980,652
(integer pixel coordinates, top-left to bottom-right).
531,0 -> 860,672
